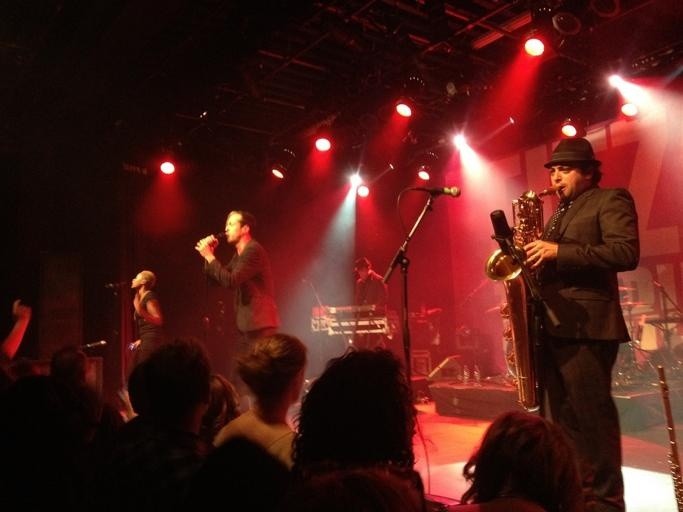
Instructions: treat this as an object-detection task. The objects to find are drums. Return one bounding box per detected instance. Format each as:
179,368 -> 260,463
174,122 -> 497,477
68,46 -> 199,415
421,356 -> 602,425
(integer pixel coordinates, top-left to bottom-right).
635,322 -> 668,350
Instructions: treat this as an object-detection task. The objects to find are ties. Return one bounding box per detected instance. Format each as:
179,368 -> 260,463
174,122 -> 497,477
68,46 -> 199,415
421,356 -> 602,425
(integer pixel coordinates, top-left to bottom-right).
544,202 -> 564,242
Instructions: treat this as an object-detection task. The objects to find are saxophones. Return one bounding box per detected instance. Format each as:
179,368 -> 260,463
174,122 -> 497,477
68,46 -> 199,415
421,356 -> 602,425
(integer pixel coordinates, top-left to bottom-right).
485,186 -> 564,416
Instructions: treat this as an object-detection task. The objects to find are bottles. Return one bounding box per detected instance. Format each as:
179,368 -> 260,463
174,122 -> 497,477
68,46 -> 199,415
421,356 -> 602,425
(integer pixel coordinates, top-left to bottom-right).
462,364 -> 470,385
472,364 -> 480,386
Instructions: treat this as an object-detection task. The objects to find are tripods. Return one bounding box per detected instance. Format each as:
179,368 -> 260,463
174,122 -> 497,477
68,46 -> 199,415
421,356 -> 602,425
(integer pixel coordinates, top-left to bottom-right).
613,310 -> 664,392
657,300 -> 683,381
448,308 -> 507,387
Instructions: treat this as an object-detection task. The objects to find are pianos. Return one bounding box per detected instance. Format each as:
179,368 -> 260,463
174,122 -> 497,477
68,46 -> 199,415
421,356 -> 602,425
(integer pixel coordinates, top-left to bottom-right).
310,305 -> 389,339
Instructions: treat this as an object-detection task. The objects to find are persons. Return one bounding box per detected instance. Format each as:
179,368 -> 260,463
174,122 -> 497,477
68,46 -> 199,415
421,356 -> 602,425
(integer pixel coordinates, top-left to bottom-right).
525,136 -> 641,512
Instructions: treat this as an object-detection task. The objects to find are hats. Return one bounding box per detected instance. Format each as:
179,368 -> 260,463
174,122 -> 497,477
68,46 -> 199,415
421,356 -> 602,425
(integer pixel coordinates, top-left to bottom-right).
543,138 -> 601,168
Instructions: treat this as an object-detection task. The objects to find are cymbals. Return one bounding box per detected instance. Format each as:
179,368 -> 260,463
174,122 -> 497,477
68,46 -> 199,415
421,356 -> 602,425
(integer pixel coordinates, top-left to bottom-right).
621,300 -> 649,306
618,284 -> 636,292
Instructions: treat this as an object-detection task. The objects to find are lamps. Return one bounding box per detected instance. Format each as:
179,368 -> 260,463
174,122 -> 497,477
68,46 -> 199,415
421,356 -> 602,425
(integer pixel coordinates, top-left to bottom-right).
352,97 -> 642,198
265,33 -> 562,181
157,150 -> 177,176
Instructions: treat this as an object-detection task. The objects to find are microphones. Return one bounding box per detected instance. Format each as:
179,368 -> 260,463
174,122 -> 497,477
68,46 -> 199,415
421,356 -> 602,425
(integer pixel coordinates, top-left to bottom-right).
651,279 -> 665,290
416,186 -> 461,198
87,340 -> 107,348
192,232 -> 225,257
